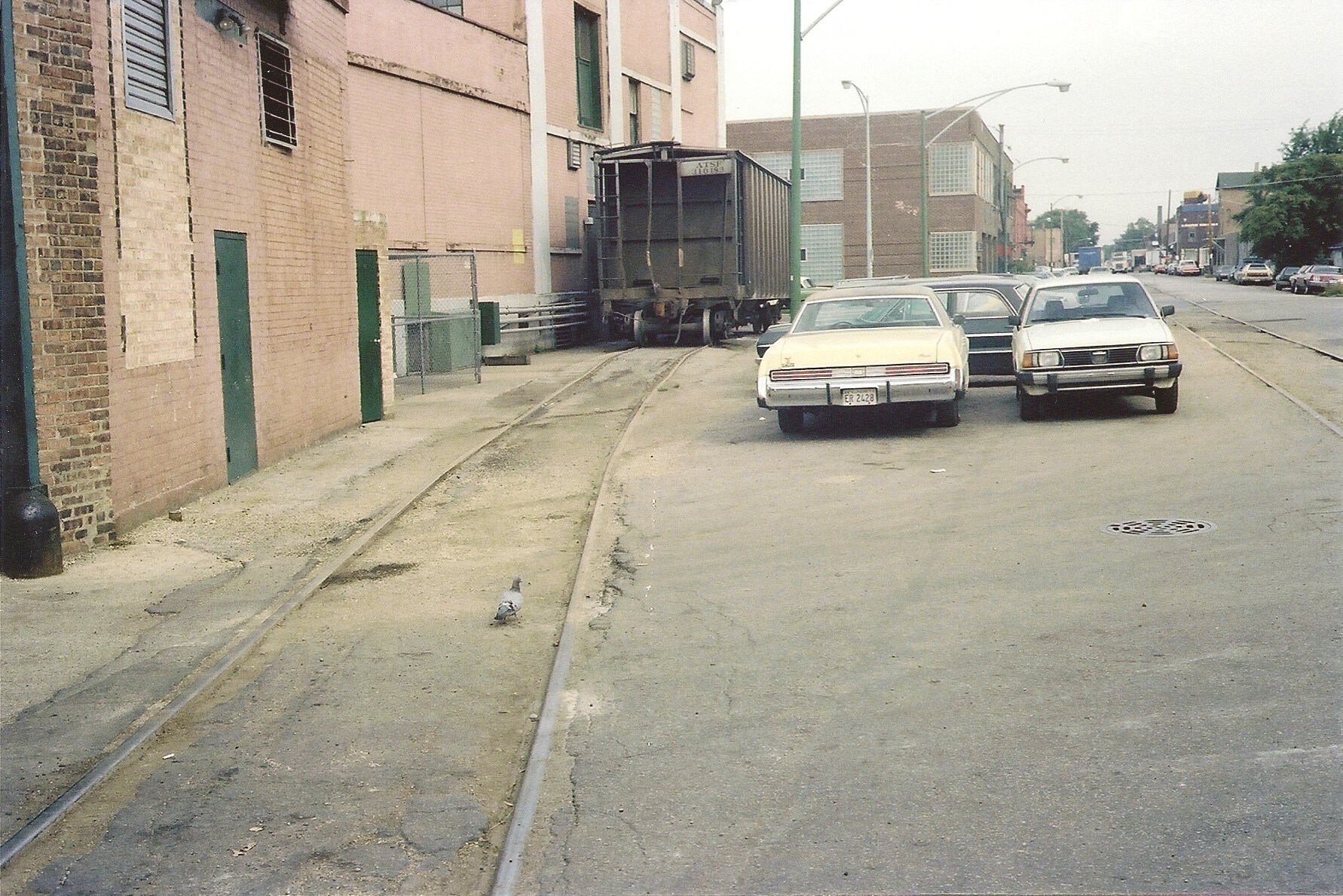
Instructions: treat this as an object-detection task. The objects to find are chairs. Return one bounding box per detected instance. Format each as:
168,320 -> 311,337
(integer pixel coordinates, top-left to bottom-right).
1107,295 -> 1129,312
915,313 -> 938,325
861,310 -> 889,322
1043,301 -> 1065,316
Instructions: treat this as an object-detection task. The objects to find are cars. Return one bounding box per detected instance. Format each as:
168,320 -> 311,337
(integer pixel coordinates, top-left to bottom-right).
756,285 -> 970,434
754,246 -> 1343,376
1006,274 -> 1182,421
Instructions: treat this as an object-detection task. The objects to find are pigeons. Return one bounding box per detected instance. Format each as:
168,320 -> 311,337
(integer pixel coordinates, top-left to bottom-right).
494,577 -> 523,624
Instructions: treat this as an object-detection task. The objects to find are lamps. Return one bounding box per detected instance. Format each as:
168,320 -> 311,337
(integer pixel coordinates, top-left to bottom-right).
217,8 -> 253,37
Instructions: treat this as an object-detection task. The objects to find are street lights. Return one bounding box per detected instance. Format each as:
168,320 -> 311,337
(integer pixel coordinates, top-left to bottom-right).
1004,157 -> 1071,274
1069,237 -> 1092,267
839,78 -> 874,277
1050,193 -> 1083,272
920,78 -> 1072,277
